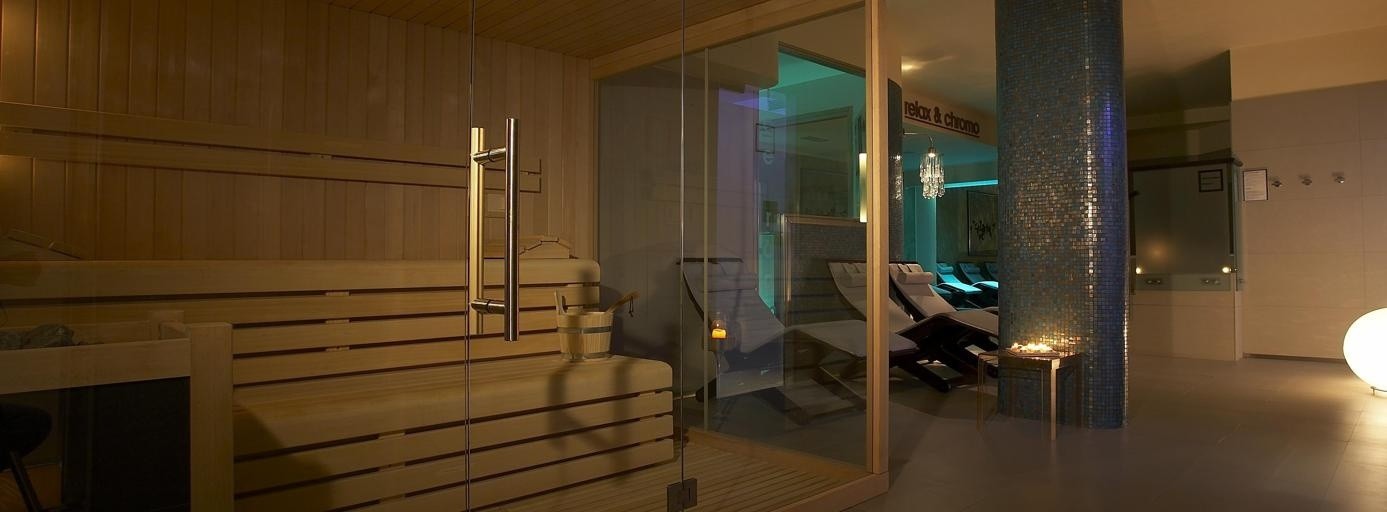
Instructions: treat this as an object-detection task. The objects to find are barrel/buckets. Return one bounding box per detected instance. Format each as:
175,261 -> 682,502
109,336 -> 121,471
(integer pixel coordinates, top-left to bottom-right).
554,291 -> 614,361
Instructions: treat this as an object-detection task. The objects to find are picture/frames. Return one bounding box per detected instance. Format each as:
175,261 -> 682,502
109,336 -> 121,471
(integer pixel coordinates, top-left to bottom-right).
964,189 -> 996,257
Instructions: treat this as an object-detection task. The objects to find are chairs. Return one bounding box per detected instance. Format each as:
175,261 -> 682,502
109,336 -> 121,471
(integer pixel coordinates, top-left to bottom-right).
674,253 -> 997,419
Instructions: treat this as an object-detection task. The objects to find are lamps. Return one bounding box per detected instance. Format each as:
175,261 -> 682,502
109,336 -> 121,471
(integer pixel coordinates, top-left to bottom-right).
901,125 -> 946,201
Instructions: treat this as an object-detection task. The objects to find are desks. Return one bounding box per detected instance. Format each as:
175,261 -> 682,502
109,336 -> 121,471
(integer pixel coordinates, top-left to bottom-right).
976,348 -> 1084,444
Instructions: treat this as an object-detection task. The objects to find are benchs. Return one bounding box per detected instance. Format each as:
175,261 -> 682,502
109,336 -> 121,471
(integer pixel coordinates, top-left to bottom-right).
0,247 -> 681,507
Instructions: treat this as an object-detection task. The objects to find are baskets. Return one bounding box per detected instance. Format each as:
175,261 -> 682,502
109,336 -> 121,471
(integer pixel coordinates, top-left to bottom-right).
557,312 -> 614,362
